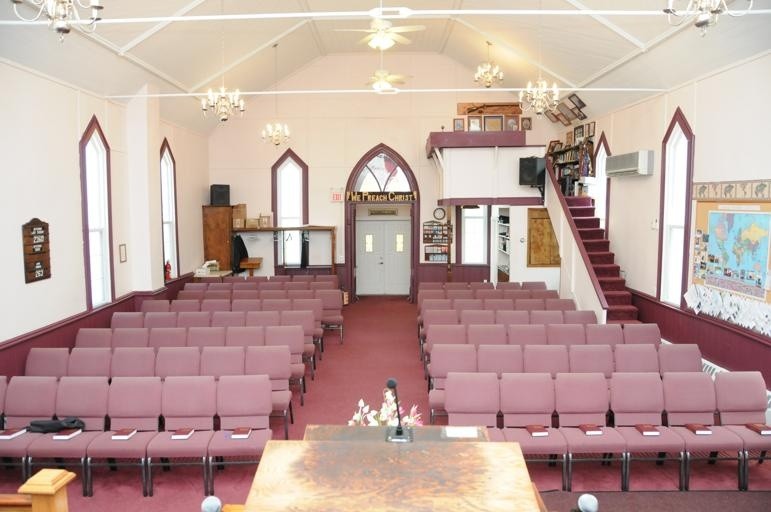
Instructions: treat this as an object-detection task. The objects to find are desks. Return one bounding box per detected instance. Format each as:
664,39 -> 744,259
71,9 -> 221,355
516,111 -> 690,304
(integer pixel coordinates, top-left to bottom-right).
242,441 -> 544,511
302,423 -> 491,441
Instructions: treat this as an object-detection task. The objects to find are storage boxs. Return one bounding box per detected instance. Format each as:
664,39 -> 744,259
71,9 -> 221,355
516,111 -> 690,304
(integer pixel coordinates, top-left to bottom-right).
245,218 -> 260,229
232,204 -> 247,228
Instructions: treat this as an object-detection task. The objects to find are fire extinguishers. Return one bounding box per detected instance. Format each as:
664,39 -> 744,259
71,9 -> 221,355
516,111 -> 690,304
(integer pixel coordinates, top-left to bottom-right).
164,260 -> 171,280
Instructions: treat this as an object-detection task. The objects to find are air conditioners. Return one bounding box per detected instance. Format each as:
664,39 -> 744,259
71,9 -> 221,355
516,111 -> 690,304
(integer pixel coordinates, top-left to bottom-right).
606,149 -> 654,178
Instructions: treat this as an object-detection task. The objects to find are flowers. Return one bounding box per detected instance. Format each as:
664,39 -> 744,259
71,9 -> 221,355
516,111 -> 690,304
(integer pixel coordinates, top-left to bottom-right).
347,388 -> 426,427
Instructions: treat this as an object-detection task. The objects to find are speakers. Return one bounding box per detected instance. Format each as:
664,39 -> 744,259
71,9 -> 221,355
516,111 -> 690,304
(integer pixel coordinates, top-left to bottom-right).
518,157 -> 544,186
210,184 -> 230,205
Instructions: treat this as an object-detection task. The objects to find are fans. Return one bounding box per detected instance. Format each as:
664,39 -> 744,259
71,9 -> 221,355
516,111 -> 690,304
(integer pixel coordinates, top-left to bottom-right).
362,50 -> 414,87
330,1 -> 427,47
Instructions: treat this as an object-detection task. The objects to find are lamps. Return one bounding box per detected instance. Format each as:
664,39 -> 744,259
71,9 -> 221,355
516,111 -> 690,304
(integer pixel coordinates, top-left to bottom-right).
201,0 -> 247,125
10,0 -> 105,41
260,43 -> 292,148
472,37 -> 504,88
518,1 -> 562,116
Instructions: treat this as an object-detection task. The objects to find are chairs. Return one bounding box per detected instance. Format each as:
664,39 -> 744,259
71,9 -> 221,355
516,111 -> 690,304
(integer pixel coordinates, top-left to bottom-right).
232,235 -> 263,276
1,274 -> 343,496
418,283 -> 769,492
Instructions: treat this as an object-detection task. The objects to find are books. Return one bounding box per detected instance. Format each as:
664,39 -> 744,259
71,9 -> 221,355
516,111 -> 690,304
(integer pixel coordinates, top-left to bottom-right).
526,425 -> 549,437
746,423 -> 771,435
171,428 -> 194,440
579,424 -> 602,436
52,428 -> 82,440
111,429 -> 137,440
635,424 -> 660,436
0,427 -> 27,440
230,427 -> 251,439
686,423 -> 712,435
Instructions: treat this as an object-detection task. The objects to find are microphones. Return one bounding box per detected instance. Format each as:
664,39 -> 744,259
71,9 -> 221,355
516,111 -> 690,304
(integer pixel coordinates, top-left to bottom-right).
386,379 -> 411,442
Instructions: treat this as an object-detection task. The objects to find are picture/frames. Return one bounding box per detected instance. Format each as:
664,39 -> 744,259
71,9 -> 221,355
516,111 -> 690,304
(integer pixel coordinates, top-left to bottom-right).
119,243 -> 127,262
452,115 -> 533,131
260,214 -> 271,228
543,122 -> 597,153
542,93 -> 588,126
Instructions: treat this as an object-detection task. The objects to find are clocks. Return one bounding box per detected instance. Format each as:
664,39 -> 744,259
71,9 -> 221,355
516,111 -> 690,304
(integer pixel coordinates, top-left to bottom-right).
434,207 -> 445,220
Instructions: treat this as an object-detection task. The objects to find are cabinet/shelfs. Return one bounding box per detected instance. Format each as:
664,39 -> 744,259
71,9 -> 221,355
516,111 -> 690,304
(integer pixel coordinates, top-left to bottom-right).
496,218 -> 511,276
423,220 -> 452,245
202,204 -> 232,273
424,244 -> 450,261
546,143 -> 594,182
